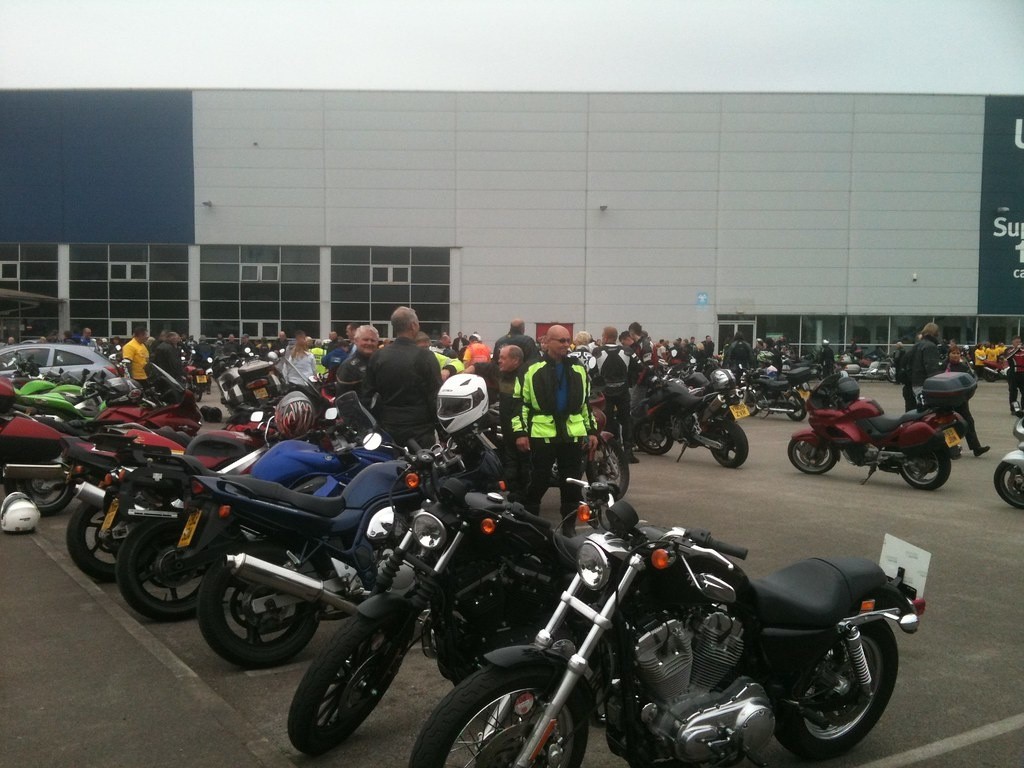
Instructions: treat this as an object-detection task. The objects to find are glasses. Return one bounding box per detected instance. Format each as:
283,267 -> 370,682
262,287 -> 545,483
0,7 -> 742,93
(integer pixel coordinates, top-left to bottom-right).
551,337 -> 571,344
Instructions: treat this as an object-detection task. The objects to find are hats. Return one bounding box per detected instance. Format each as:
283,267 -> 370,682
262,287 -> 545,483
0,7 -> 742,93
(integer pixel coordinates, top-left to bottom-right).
241,333 -> 249,339
822,340 -> 830,345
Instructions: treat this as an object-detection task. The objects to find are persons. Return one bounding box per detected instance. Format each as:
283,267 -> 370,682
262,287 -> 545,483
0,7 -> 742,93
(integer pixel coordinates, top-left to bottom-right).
509,324 -> 598,538
0,319 -> 1024,466
361,307 -> 439,455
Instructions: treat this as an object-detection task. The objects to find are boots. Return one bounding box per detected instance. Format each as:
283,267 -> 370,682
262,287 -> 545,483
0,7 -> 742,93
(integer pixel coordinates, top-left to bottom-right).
624,440 -> 639,463
526,502 -> 540,517
560,501 -> 577,538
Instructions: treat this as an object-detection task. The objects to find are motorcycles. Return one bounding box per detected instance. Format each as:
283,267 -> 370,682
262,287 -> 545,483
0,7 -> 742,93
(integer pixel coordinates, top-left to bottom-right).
737,343 -> 1011,422
787,371 -> 978,492
109,343 -> 285,421
548,392 -> 631,502
409,458 -> 934,768
174,422 -> 508,670
631,349 -> 751,468
286,437 -> 672,756
96,390 -> 402,622
58,385 -> 332,584
1,349 -> 202,519
994,401 -> 1024,509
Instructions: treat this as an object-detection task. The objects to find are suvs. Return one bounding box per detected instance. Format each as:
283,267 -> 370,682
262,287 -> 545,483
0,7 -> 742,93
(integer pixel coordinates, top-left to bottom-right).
1,344 -> 128,393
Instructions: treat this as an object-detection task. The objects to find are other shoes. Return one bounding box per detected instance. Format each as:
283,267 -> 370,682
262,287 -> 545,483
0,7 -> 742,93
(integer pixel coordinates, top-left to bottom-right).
1010,409 -> 1017,415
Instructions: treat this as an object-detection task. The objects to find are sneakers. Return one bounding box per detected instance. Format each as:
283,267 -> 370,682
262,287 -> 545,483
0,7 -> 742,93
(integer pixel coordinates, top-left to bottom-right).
972,446 -> 990,456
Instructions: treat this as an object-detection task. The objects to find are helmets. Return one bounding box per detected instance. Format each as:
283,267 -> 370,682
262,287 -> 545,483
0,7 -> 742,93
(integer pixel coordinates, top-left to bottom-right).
274,391 -> 315,438
756,350 -> 774,364
1,492 -> 41,532
437,373 -> 488,433
103,377 -> 143,407
963,344 -> 968,350
